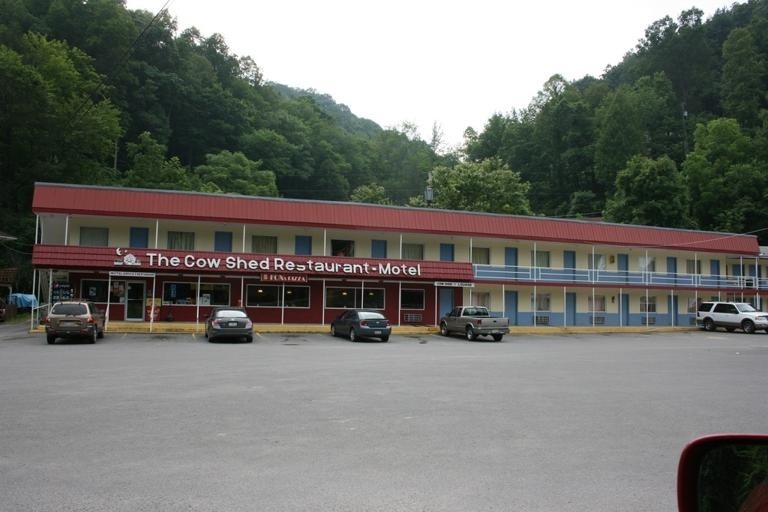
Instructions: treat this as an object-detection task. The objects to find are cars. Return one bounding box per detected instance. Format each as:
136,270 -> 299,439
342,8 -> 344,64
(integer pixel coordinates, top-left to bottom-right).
204,307 -> 253,343
329,310 -> 390,342
44,299 -> 105,344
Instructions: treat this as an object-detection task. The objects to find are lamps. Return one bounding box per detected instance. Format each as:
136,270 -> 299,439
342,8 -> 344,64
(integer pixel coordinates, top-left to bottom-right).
424,186 -> 433,208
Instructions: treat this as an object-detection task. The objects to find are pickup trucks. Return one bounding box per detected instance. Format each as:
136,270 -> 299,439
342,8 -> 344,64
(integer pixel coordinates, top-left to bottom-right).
439,305 -> 510,342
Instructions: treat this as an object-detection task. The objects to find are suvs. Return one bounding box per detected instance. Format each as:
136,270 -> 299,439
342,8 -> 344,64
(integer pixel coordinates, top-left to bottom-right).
696,298 -> 767,333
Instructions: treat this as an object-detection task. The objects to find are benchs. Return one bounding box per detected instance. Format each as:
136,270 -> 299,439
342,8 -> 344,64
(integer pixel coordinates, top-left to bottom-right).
642,316 -> 655,326
592,317 -> 605,325
533,316 -> 549,325
